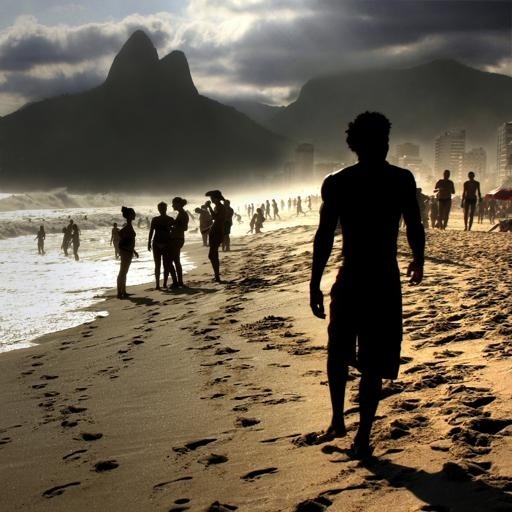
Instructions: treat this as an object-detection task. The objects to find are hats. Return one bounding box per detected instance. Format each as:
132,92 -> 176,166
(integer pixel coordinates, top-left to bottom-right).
205,190 -> 225,201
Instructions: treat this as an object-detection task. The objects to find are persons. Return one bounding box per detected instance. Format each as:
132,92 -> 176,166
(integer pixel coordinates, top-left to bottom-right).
309,110 -> 425,459
33,170 -> 512,298
